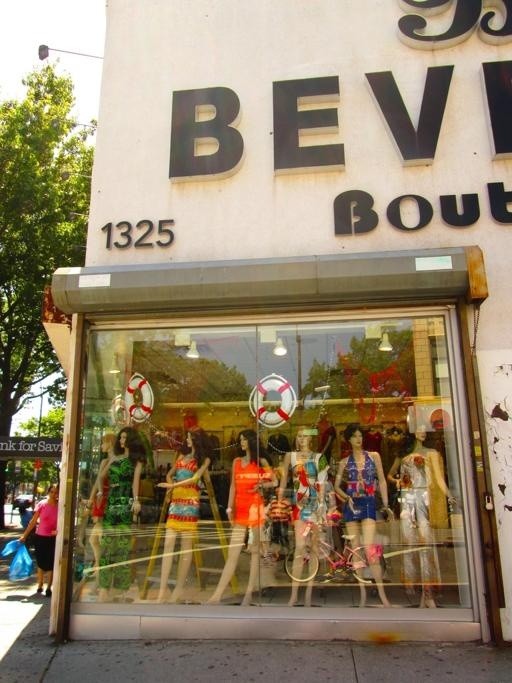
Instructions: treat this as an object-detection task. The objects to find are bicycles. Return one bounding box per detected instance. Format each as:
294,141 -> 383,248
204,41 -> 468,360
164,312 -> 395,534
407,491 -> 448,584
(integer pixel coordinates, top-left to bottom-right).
284,520 -> 387,583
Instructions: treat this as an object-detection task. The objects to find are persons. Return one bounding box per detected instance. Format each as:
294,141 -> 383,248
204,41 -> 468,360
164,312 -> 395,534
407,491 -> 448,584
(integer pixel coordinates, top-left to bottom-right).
388,419 -> 456,609
207,429 -> 279,606
154,428 -> 211,604
278,424 -> 330,610
84,426 -> 146,604
18,483 -> 59,598
334,423 -> 389,610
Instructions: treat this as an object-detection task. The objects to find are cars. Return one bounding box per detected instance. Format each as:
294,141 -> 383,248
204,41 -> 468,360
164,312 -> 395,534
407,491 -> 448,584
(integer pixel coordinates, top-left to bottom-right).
12,494 -> 39,510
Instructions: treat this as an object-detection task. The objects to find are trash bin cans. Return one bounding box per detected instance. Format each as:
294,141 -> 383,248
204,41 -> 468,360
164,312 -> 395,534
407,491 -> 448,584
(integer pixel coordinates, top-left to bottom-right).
21,511 -> 36,532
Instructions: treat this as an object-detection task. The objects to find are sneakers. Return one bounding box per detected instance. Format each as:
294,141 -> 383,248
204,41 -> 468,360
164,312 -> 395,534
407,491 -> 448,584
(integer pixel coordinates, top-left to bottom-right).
38,588 -> 51,597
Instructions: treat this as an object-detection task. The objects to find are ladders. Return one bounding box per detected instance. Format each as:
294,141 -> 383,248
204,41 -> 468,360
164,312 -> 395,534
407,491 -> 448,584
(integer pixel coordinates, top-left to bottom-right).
139,449 -> 241,599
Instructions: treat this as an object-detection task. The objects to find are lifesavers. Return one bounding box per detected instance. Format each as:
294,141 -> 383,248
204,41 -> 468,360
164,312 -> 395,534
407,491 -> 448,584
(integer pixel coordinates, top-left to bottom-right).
125,377 -> 151,418
253,379 -> 293,423
